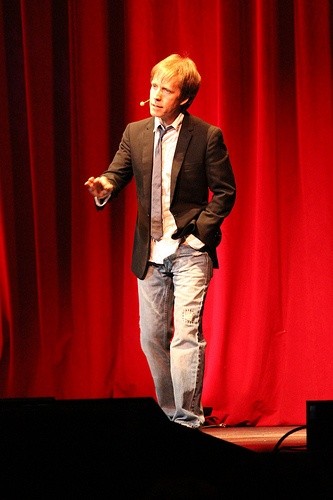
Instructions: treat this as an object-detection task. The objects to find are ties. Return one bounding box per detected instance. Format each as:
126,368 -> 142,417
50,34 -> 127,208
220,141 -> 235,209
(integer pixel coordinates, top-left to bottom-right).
151,125 -> 174,241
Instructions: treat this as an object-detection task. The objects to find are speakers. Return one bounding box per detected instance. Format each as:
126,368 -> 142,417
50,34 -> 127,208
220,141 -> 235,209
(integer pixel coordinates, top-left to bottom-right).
0,396 -> 180,500
306,400 -> 333,465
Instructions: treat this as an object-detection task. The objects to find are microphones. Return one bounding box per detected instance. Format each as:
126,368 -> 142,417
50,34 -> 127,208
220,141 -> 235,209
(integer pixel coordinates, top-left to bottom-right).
140,99 -> 150,106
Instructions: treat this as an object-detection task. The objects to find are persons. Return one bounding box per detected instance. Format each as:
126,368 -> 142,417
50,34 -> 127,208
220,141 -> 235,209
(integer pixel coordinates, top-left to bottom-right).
84,55 -> 236,429
